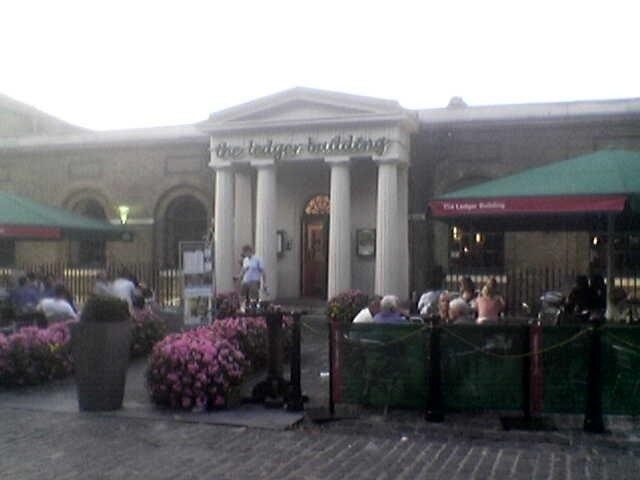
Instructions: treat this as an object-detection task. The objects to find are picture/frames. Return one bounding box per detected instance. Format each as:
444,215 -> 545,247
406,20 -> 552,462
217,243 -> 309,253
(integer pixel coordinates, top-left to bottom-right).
277,228 -> 284,256
355,227 -> 378,259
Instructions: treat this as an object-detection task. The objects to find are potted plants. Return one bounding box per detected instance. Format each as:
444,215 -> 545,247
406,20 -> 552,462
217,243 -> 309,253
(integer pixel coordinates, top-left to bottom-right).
65,295 -> 133,415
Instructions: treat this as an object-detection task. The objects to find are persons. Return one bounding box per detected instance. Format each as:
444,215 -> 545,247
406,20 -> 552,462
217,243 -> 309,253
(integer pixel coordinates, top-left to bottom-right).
352,275 -> 633,324
240,245 -> 268,301
0,266 -> 151,327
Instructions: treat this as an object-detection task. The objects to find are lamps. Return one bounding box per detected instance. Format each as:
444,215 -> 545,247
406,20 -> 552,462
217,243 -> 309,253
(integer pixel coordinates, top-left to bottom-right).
118,204 -> 130,226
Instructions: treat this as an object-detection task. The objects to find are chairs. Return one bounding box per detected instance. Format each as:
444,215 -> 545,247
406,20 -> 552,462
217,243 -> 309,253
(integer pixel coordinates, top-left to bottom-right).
1,292 -> 47,333
396,299 -> 635,327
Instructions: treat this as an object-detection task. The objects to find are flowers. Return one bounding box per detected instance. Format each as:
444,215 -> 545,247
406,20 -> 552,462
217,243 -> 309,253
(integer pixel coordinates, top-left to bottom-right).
326,291 -> 382,327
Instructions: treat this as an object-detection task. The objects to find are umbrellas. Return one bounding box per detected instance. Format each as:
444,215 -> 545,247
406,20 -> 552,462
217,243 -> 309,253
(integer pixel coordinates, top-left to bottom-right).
424,146 -> 640,320
0,189 -> 133,242
